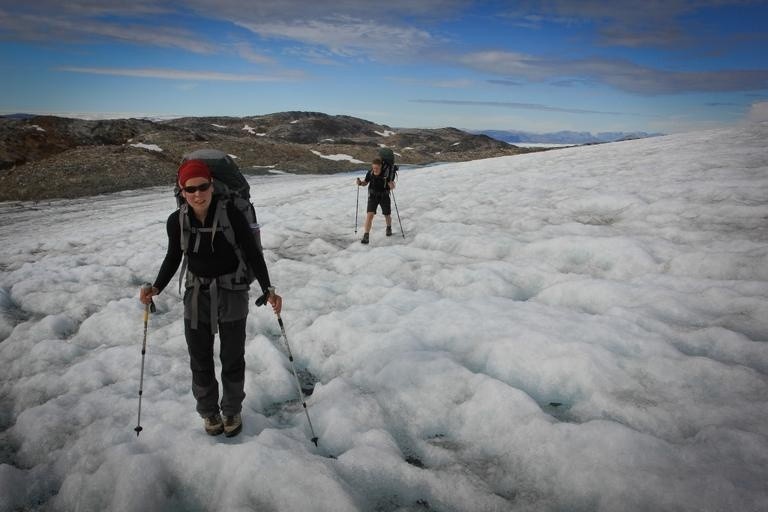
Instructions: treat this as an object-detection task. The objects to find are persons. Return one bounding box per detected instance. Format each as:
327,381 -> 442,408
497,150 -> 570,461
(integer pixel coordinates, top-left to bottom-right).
139,160 -> 283,438
356,158 -> 395,244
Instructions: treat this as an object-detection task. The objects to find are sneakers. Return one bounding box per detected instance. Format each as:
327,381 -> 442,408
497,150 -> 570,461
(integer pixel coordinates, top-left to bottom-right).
385,227 -> 392,236
204,412 -> 224,436
361,233 -> 369,244
223,412 -> 242,437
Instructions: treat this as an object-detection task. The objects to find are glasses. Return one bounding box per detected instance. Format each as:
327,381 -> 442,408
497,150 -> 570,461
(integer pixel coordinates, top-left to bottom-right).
180,179 -> 211,194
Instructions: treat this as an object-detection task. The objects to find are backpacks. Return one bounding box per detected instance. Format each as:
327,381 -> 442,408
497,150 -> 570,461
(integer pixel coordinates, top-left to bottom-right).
175,149 -> 261,292
370,148 -> 396,191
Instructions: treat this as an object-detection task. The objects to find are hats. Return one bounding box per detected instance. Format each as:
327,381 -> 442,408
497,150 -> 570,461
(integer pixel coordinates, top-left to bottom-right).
178,159 -> 209,187
